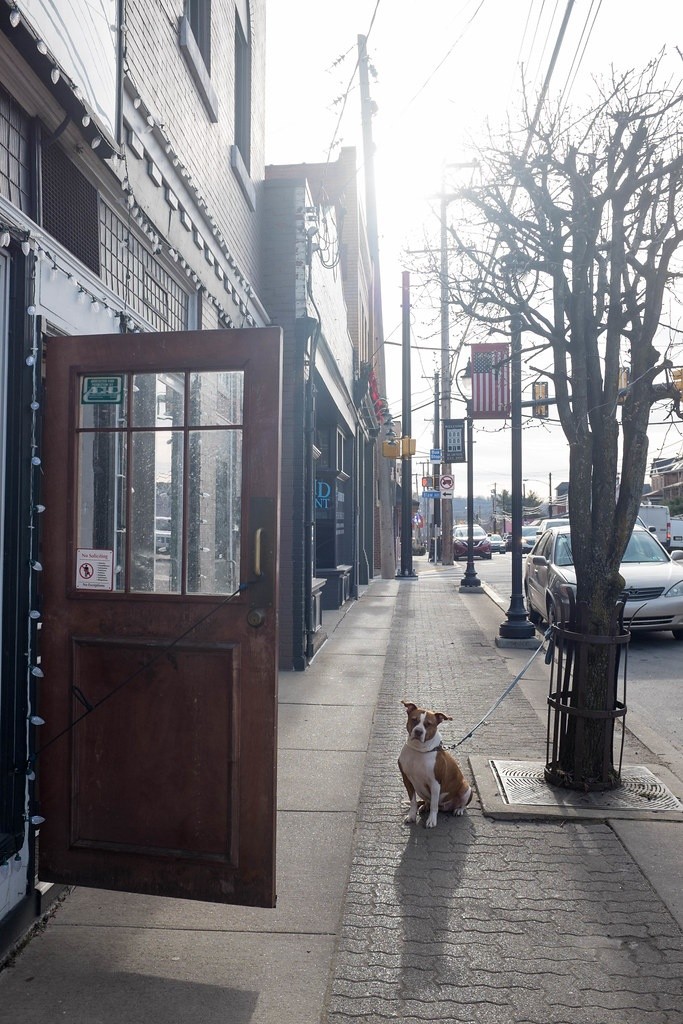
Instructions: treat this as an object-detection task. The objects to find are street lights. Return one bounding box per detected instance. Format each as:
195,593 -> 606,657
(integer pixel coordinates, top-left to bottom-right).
523,478 -> 553,519
455,355 -> 482,593
496,249 -> 538,649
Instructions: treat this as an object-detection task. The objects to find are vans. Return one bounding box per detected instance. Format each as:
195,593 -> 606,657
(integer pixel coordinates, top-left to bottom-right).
638,505 -> 671,550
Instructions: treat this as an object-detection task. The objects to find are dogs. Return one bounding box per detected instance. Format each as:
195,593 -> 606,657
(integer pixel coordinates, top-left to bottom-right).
396,700 -> 473,829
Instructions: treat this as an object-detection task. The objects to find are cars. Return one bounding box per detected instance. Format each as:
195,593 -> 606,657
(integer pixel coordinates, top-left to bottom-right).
522,526 -> 541,553
523,523 -> 683,642
488,534 -> 506,554
536,519 -> 569,540
453,524 -> 492,561
502,534 -> 514,551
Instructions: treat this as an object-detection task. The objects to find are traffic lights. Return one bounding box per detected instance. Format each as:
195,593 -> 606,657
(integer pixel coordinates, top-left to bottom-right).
422,477 -> 430,488
